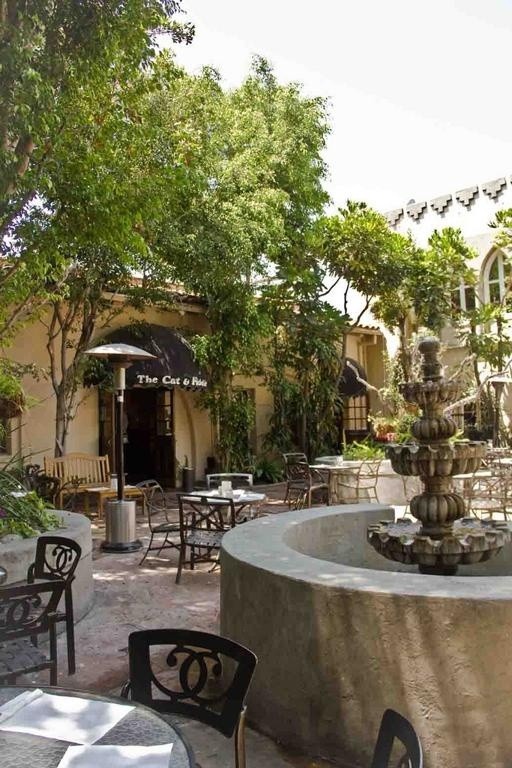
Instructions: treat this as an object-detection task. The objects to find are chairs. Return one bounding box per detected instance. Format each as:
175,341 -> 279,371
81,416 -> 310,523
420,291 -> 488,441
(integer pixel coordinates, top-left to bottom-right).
136,472 -> 254,585
281,452 -> 384,511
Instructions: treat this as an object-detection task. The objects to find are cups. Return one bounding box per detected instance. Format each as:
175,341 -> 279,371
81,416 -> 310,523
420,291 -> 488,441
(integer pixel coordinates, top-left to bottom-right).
221,481 -> 232,498
218,485 -> 222,496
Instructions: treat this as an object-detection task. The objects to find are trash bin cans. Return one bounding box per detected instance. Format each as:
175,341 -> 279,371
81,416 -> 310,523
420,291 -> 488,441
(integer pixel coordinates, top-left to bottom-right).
183,467 -> 195,491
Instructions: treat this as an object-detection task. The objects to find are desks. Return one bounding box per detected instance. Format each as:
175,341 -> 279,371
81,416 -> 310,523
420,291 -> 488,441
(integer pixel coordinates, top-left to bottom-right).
80,480 -> 150,520
178,489 -> 266,547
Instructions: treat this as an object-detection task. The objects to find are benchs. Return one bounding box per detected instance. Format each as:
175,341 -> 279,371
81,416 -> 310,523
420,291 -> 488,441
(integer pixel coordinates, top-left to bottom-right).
39,451 -> 112,515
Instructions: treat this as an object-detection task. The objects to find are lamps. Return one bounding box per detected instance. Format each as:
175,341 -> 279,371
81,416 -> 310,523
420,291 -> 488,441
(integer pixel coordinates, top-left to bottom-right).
82,340 -> 157,554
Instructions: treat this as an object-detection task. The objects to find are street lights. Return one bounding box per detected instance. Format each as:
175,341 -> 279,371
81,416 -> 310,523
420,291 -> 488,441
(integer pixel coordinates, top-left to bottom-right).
82,344 -> 158,500
